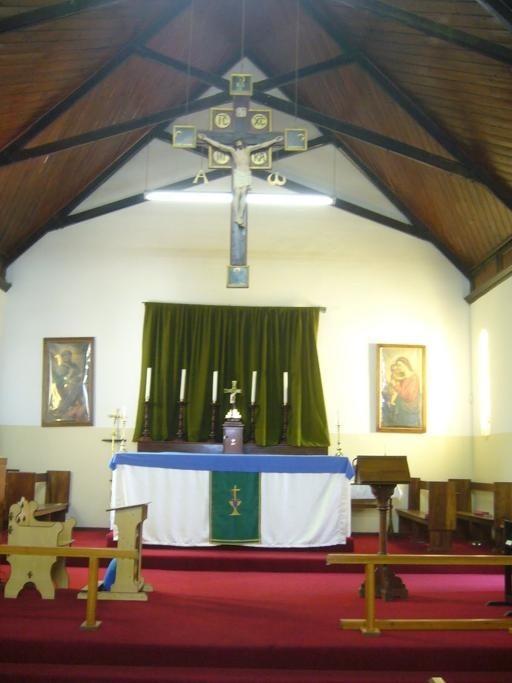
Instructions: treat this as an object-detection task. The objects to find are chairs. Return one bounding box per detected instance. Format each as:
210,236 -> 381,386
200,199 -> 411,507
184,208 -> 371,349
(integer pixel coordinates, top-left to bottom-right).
5,499 -> 76,602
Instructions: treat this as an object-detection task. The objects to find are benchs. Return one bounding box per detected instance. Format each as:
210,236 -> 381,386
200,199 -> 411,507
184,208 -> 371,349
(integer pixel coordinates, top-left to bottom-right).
396,474 -> 512,551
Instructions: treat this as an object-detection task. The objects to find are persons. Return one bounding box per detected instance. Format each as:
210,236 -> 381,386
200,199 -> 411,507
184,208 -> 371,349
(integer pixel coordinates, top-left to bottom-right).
56,350 -> 82,416
382,357 -> 419,426
197,133 -> 284,228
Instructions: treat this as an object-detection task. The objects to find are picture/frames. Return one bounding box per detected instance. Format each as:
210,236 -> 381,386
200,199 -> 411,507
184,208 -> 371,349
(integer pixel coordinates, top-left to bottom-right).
375,342 -> 429,436
41,335 -> 95,427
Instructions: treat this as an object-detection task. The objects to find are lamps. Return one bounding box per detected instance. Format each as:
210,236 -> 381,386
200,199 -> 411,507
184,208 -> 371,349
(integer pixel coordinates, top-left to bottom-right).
351,454 -> 412,601
